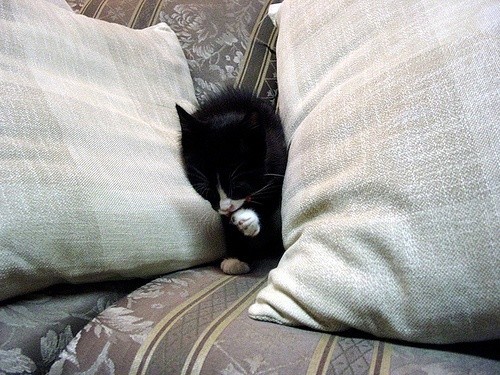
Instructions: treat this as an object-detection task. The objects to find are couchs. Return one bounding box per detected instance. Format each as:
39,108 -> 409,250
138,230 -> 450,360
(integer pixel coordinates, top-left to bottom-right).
0,1 -> 500,375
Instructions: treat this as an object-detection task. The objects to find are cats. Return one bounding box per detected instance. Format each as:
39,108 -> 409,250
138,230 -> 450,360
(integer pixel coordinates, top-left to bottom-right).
175,85 -> 288,276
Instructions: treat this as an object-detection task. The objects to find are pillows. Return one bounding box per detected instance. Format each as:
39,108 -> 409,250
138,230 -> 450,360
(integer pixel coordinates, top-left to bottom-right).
246,1 -> 500,344
0,1 -> 233,300
